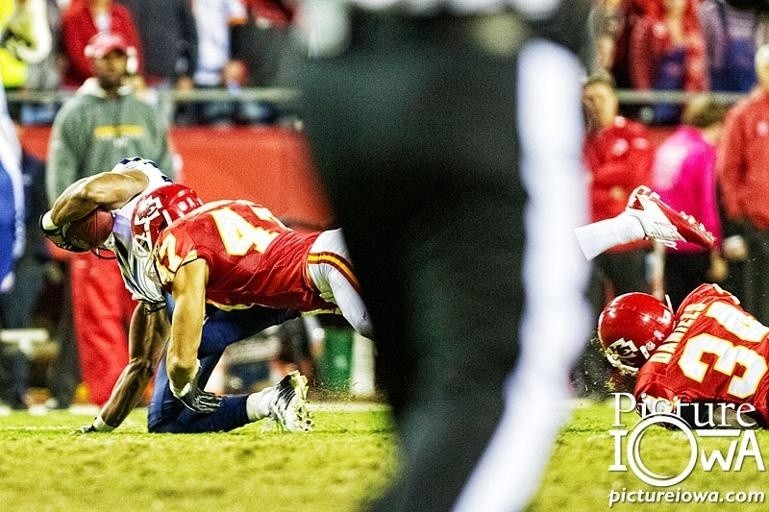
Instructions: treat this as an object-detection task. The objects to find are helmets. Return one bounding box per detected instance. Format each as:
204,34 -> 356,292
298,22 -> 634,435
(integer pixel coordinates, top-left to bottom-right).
111,157 -> 158,171
598,292 -> 679,379
84,30 -> 130,60
131,183 -> 203,259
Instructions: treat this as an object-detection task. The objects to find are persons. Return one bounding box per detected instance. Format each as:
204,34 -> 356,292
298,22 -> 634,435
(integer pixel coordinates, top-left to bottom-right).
0,0 -> 769,512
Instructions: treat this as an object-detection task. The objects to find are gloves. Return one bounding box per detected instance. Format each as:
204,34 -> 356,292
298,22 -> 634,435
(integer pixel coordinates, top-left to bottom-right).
73,416 -> 115,433
38,209 -> 91,252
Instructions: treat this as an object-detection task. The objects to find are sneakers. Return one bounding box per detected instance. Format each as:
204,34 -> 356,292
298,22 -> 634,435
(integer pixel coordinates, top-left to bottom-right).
273,369 -> 313,433
626,185 -> 716,249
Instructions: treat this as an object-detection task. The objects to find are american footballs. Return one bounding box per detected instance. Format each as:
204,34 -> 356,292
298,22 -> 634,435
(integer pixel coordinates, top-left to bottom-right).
70,206 -> 114,248
